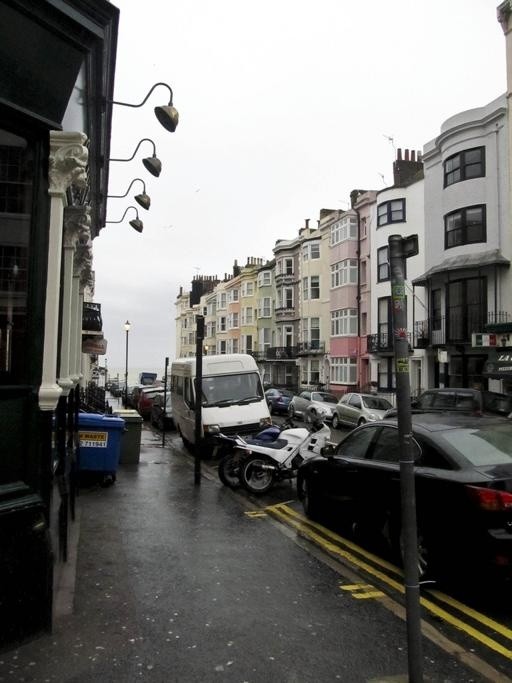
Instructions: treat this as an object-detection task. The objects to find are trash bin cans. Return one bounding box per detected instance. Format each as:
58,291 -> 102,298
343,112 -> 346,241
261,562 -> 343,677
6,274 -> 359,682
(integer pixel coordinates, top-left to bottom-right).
112,409 -> 143,464
78,413 -> 125,488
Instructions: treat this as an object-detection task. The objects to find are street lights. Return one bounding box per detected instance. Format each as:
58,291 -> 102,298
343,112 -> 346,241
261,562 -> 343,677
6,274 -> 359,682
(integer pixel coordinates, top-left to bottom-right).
124,320 -> 131,409
